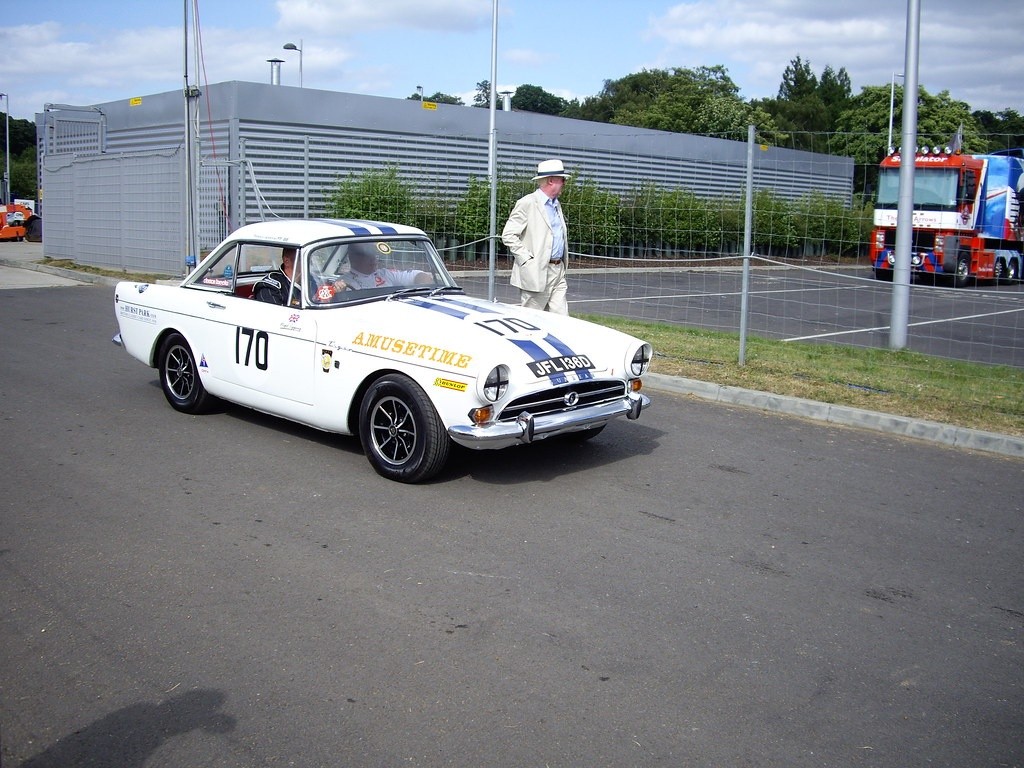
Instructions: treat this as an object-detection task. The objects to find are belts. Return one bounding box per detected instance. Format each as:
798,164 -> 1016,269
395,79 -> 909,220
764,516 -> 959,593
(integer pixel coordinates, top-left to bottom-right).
549,259 -> 563,265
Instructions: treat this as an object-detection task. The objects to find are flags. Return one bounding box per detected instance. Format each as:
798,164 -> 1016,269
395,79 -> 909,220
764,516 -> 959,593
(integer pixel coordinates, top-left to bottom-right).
948,125 -> 962,153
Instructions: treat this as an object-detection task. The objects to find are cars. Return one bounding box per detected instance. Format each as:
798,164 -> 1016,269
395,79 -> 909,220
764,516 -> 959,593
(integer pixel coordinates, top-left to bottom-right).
112,217 -> 652,485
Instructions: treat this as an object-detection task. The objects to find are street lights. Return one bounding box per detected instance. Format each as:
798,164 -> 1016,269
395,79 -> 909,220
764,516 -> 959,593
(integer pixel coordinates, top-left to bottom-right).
283,38 -> 303,89
417,85 -> 423,101
887,70 -> 904,157
0,92 -> 10,205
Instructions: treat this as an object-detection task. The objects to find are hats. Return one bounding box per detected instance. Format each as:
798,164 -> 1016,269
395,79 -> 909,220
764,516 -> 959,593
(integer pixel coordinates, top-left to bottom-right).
531,160 -> 572,181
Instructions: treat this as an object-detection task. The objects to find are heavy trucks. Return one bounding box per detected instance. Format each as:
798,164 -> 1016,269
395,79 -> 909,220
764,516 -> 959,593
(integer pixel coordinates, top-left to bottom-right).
869,145 -> 1024,287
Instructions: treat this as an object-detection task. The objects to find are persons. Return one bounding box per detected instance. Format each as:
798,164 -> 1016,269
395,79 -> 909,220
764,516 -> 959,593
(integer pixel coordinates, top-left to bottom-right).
502,160 -> 570,316
334,243 -> 433,290
252,248 -> 346,306
